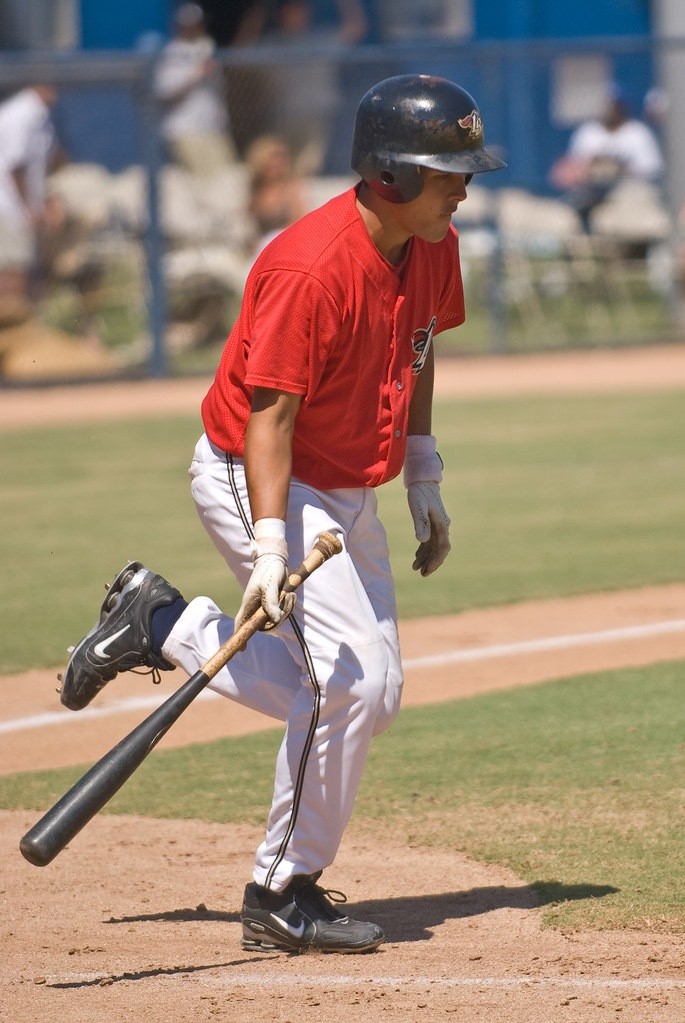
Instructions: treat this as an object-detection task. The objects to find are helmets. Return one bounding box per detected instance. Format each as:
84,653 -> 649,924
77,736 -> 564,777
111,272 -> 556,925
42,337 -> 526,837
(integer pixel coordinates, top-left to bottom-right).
349,73 -> 507,203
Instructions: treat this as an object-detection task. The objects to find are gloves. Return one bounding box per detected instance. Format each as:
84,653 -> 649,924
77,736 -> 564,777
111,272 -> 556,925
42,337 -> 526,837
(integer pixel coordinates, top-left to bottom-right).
233,517 -> 296,652
403,435 -> 452,578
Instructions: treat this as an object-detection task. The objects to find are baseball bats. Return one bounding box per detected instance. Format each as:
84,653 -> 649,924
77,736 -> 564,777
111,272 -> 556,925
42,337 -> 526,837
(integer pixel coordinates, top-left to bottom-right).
20,533 -> 344,868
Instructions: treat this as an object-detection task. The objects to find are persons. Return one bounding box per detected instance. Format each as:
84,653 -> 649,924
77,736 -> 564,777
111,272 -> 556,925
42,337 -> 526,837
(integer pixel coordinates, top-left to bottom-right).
56,73 -> 508,954
0,0 -> 675,385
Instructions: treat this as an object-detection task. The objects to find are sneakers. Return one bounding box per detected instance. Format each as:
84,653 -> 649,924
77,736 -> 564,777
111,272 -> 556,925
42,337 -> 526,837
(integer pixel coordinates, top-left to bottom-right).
239,869 -> 385,954
54,560 -> 182,711
